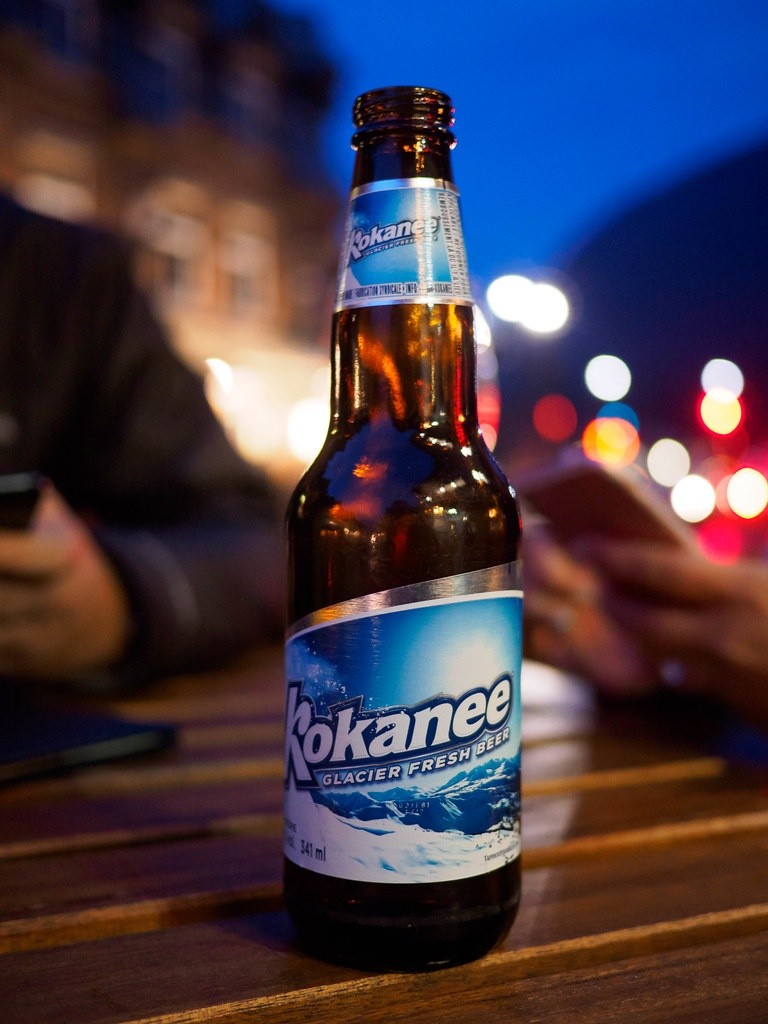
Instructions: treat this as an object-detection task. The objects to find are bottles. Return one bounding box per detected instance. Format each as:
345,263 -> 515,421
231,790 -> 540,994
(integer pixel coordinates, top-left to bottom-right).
279,86 -> 523,971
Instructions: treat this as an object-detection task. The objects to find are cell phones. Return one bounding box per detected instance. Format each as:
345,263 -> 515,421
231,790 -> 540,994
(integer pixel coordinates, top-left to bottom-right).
515,460 -> 707,565
0,707 -> 177,784
0,473 -> 41,530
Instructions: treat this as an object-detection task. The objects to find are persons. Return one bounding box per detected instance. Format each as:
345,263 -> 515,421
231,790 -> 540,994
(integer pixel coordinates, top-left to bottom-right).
1,0 -> 768,723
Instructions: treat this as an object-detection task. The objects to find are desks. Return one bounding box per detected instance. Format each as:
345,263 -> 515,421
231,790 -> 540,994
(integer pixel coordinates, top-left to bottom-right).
1,647 -> 767,1024
